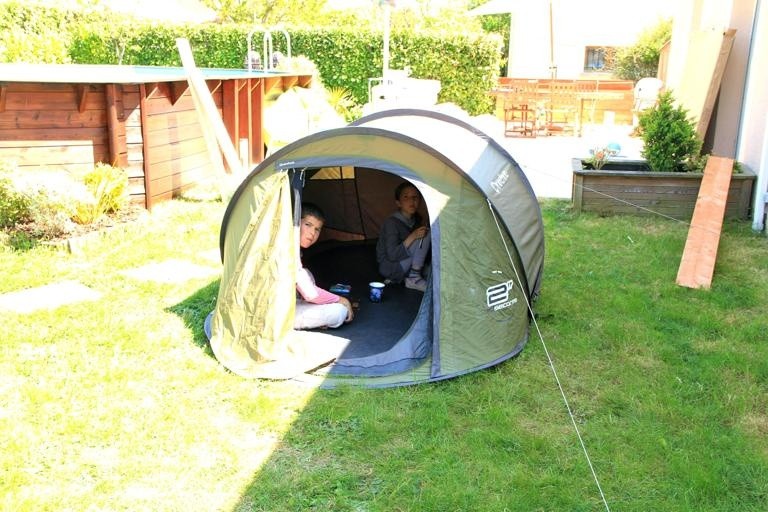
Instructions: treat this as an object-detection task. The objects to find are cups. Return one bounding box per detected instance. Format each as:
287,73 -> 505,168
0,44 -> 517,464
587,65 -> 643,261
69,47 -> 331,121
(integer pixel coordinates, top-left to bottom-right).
369,282 -> 385,302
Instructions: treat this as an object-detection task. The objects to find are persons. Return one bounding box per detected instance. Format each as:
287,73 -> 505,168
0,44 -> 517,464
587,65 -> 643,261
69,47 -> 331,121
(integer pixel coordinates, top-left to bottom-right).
376,181 -> 431,292
293,202 -> 355,330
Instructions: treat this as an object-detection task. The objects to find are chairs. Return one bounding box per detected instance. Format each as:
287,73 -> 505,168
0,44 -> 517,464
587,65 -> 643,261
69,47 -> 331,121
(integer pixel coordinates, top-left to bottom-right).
504,77 -> 598,140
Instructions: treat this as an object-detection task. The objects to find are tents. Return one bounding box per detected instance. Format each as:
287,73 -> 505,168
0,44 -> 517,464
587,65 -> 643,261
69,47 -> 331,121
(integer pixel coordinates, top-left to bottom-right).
204,108 -> 545,391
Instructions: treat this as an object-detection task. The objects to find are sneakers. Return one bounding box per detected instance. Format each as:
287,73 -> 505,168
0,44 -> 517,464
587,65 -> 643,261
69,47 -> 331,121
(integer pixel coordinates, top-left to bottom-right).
405,275 -> 427,292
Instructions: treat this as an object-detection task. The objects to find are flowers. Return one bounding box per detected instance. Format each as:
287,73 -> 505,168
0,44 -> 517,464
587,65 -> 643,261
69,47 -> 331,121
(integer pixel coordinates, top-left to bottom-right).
583,147 -> 617,170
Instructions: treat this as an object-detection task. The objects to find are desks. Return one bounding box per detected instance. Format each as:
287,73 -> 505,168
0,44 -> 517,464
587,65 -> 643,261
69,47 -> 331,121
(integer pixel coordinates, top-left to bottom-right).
488,88 -> 605,136
